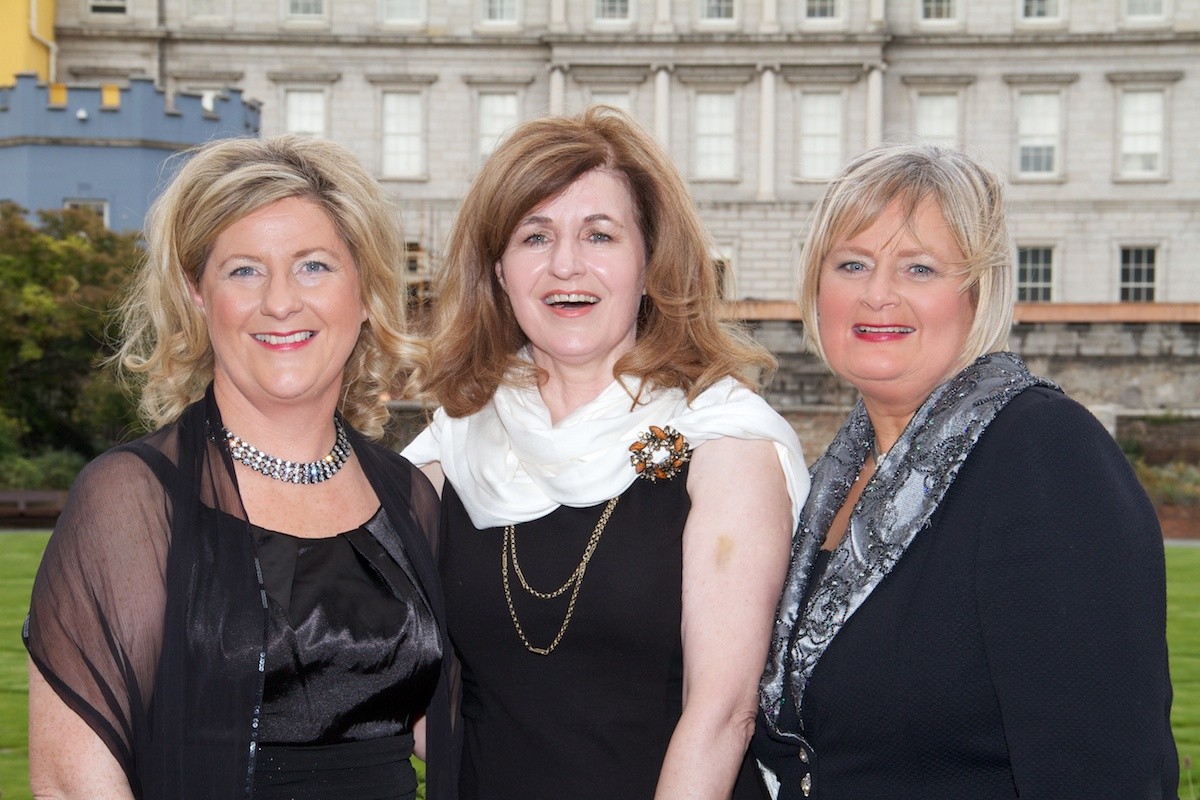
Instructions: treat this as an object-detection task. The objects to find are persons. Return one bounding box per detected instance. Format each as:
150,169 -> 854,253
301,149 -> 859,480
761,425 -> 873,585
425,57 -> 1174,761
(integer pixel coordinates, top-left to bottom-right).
21,130 -> 460,800
746,140 -> 1180,800
398,106 -> 811,799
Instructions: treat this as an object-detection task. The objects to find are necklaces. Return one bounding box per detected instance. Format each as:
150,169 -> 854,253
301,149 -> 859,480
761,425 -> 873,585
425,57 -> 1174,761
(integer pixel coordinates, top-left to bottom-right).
871,435 -> 886,471
503,495 -> 619,654
205,417 -> 352,484
